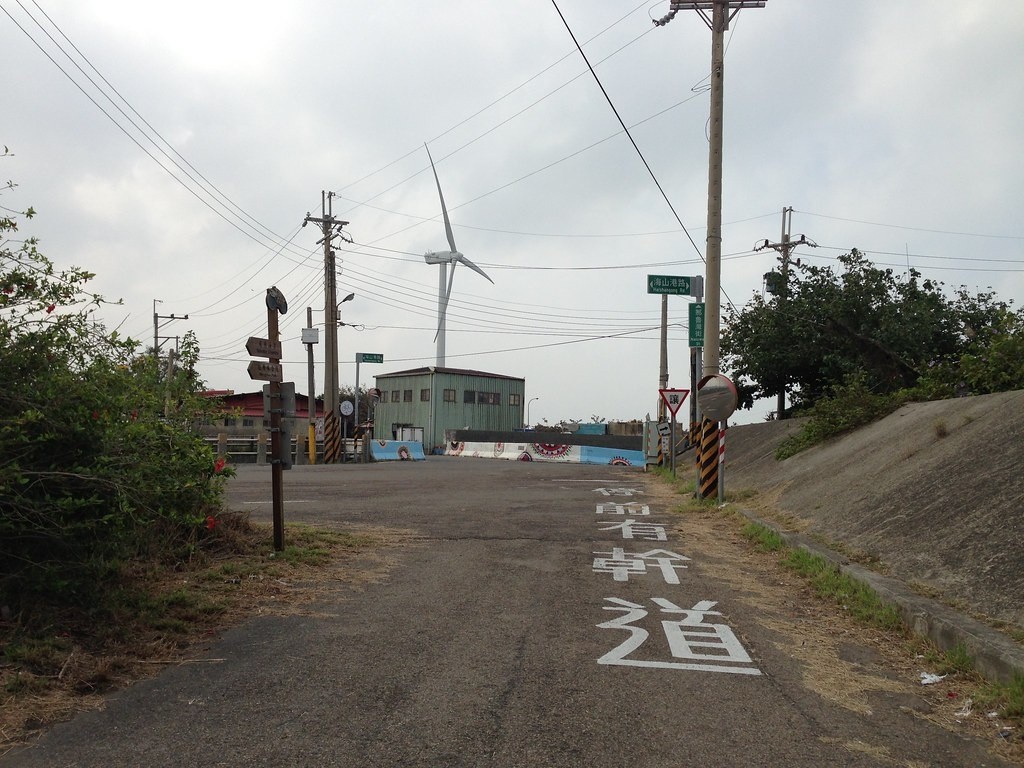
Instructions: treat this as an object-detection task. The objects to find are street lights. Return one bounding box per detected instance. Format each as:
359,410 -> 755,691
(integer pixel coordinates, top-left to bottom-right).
332,291 -> 355,464
527,397 -> 538,432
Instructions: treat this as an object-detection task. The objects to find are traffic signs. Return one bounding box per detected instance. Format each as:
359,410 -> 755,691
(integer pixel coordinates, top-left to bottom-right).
245,336 -> 283,359
247,361 -> 284,382
